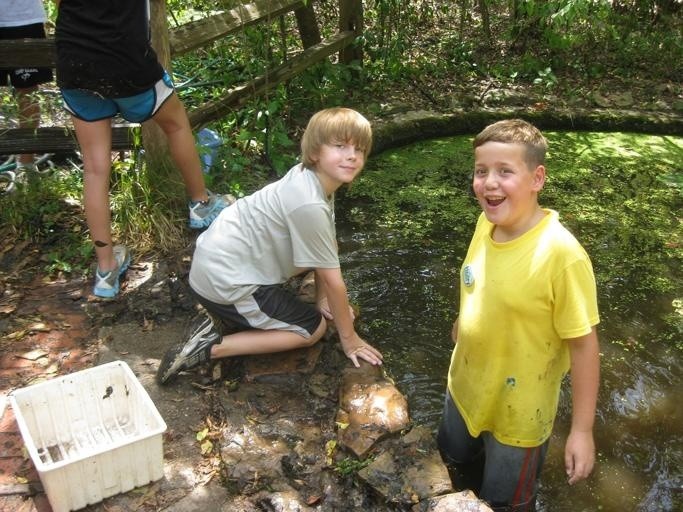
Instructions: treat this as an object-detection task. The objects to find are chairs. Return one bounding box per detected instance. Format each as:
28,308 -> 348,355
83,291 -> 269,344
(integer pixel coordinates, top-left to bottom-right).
187,187 -> 237,230
158,313 -> 223,387
92,245 -> 130,298
13,164 -> 40,187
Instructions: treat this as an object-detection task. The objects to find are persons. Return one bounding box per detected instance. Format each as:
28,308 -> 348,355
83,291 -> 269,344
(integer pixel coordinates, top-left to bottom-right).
435,117 -> 601,510
0,1 -> 52,189
154,107 -> 385,391
45,1 -> 239,301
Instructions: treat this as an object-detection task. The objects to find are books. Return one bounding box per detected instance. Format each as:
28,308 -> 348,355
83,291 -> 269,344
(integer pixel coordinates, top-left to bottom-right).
8,359 -> 168,512
